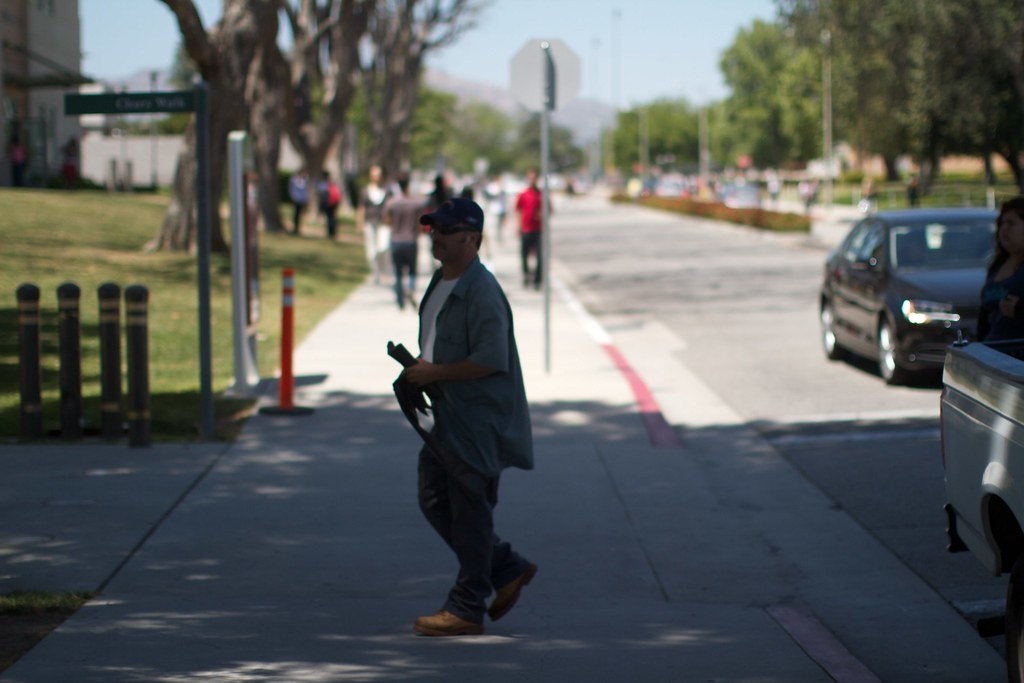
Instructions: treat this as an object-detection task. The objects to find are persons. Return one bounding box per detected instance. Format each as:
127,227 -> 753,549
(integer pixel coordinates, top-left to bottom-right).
401,197 -> 539,634
512,167 -> 554,288
289,156 -> 507,309
628,166 -> 926,215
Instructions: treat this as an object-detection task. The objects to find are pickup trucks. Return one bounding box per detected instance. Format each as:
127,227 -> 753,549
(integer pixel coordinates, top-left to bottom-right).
942,331 -> 1024,683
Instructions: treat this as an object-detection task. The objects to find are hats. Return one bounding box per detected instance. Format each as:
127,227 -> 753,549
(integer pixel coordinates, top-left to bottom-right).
420,198 -> 484,232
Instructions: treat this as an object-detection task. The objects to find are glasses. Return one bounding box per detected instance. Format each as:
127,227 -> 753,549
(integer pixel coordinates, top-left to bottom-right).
429,221 -> 478,235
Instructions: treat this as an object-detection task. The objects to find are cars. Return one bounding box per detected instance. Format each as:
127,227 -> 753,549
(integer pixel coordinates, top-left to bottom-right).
820,208 -> 1005,387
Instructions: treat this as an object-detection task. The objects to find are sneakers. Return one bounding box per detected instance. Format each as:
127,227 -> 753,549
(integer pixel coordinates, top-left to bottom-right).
488,563 -> 537,622
413,610 -> 485,636
